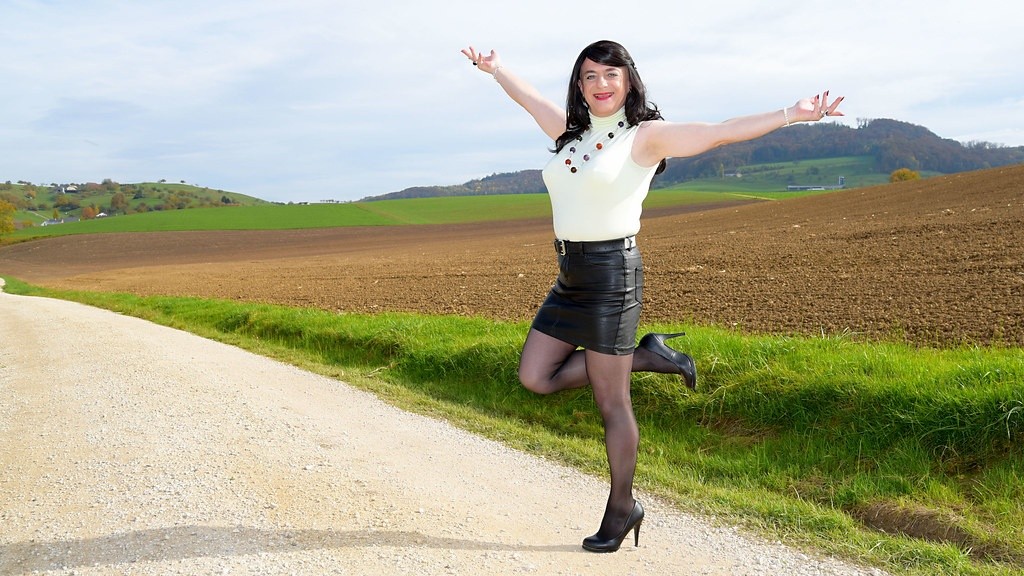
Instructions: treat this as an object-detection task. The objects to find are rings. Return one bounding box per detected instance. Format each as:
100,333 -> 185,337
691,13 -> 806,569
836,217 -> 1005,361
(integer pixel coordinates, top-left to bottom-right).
823,109 -> 828,117
473,60 -> 479,65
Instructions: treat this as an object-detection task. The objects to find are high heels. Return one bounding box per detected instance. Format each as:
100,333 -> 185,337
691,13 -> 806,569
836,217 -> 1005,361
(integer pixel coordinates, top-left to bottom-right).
639,332 -> 696,389
582,500 -> 644,553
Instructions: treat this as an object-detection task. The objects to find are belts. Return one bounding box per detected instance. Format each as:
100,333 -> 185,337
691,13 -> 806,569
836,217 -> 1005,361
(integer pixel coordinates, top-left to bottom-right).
557,234 -> 636,256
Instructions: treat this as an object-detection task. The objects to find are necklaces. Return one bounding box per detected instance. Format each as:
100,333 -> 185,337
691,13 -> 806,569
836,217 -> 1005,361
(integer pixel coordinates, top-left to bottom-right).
564,118 -> 627,173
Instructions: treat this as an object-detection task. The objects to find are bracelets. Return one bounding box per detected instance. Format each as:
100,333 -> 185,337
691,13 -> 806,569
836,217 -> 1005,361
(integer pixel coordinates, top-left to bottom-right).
492,66 -> 505,78
783,108 -> 791,127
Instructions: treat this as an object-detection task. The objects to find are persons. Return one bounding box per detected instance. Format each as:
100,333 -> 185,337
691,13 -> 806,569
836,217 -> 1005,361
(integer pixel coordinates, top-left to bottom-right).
460,40 -> 845,552
